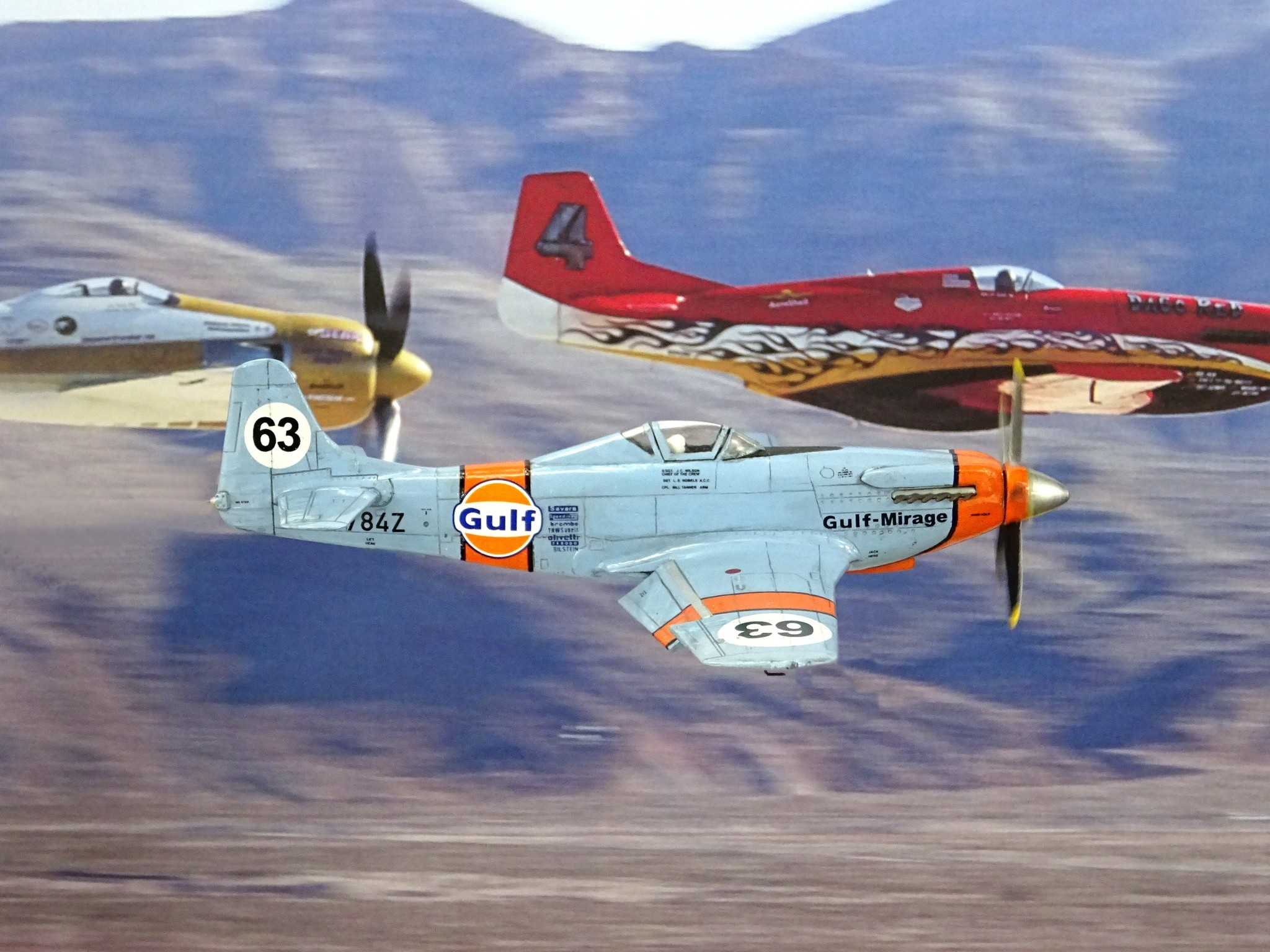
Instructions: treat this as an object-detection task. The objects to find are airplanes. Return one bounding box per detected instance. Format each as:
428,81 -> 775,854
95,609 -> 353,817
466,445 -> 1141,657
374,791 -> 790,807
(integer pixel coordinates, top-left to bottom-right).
211,357 -> 1071,664
0,229 -> 430,461
497,166 -> 1270,433
